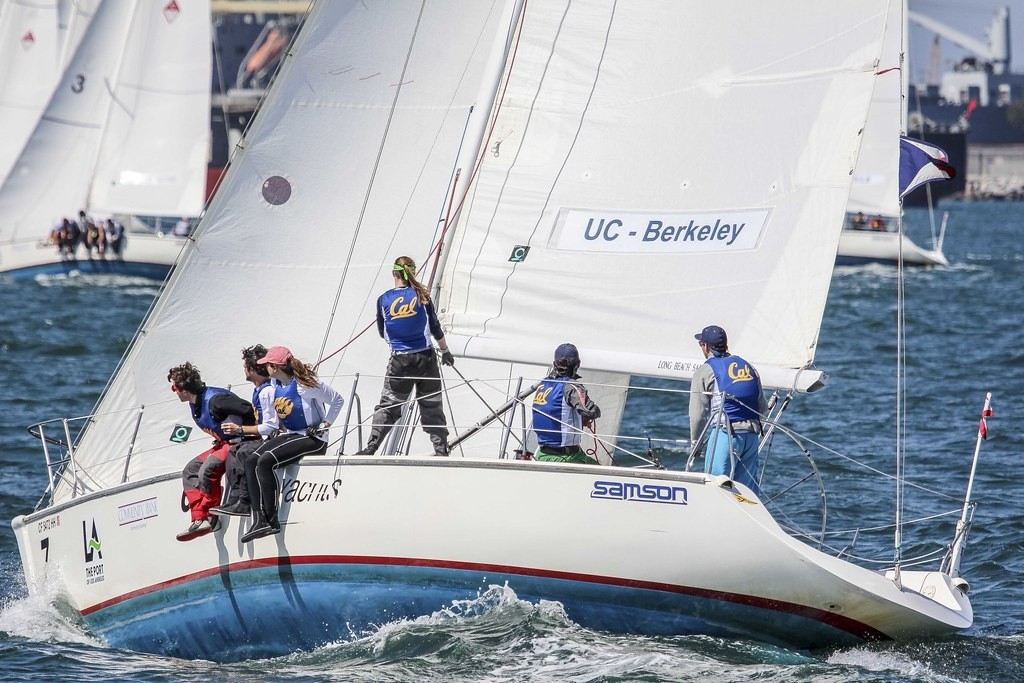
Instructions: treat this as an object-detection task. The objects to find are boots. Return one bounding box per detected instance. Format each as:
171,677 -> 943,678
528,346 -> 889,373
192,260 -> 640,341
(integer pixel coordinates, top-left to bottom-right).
240,509 -> 271,543
257,511 -> 280,538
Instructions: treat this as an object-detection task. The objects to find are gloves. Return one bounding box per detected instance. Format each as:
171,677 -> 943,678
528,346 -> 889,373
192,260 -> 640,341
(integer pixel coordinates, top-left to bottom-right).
306,423 -> 328,438
441,347 -> 454,366
691,445 -> 703,457
272,429 -> 283,438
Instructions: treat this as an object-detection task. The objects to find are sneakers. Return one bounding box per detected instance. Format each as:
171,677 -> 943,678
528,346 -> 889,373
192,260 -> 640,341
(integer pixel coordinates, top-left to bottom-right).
176,518 -> 213,541
207,516 -> 221,532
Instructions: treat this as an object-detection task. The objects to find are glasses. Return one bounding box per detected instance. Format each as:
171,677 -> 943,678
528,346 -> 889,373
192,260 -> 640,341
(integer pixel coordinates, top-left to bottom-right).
171,385 -> 177,392
699,341 -> 708,347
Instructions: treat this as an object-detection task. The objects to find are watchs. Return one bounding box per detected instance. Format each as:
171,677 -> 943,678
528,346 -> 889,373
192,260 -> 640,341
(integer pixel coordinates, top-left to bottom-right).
236,426 -> 244,435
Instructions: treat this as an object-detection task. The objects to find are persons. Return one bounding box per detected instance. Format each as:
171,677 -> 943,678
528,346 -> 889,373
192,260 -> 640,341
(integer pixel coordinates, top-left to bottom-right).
58,220 -> 81,260
689,327 -> 769,499
165,217 -> 191,237
80,209 -> 94,234
851,211 -> 866,230
533,344 -> 603,466
167,362 -> 257,542
241,346 -> 344,544
209,344 -> 284,516
867,214 -> 884,231
85,222 -> 106,260
102,218 -> 124,260
354,257 -> 455,457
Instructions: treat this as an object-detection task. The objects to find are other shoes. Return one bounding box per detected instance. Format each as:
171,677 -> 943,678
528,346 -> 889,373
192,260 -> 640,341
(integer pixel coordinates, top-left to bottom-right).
433,451 -> 448,456
209,499 -> 238,516
354,445 -> 377,455
218,497 -> 251,516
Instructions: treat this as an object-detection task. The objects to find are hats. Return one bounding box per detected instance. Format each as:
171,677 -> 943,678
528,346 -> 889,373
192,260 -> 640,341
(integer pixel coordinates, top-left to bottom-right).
257,346 -> 293,365
694,325 -> 727,348
554,344 -> 579,369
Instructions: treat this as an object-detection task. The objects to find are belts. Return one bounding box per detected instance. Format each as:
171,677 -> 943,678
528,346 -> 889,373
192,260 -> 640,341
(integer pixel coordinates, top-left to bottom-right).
540,445 -> 579,455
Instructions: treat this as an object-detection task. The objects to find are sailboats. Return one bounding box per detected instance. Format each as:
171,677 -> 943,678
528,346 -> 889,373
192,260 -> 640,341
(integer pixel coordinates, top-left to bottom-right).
0,0 -> 995,667
